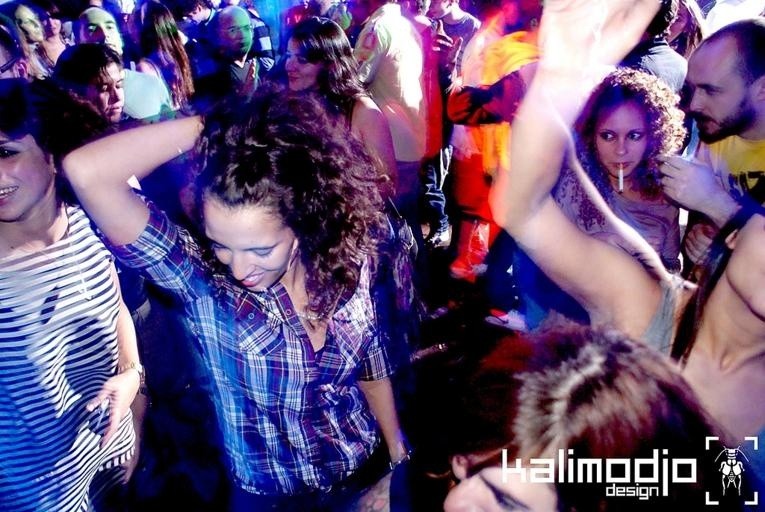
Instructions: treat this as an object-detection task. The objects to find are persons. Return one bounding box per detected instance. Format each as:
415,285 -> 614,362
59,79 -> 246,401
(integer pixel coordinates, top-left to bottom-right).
2,1 -> 764,511
